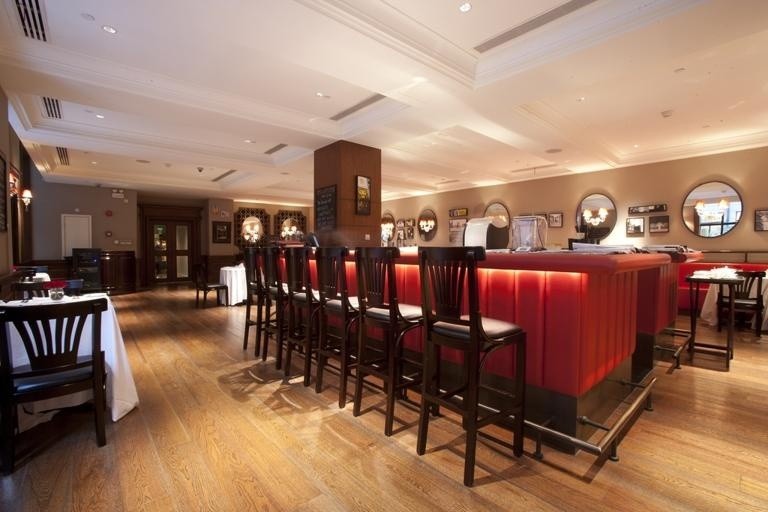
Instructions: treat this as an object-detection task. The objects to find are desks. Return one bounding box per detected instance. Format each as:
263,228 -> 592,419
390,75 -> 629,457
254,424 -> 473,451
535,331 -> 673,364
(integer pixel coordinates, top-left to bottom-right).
219,265 -> 247,306
0,273 -> 139,472
684,276 -> 744,367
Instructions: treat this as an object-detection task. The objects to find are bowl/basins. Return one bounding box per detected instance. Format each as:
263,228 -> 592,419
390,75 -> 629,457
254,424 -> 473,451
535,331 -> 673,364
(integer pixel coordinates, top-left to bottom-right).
49,287 -> 64,299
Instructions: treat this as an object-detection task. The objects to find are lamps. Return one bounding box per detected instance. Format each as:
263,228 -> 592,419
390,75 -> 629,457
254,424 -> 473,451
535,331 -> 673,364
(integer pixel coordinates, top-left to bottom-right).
19,189 -> 33,211
111,188 -> 125,199
9,170 -> 19,196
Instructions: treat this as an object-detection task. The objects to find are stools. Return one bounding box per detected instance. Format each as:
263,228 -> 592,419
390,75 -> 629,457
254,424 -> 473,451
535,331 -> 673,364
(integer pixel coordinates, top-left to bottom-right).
313,244 -> 369,410
353,245 -> 437,438
257,247 -> 307,370
281,246 -> 341,387
241,246 -> 289,357
416,244 -> 526,485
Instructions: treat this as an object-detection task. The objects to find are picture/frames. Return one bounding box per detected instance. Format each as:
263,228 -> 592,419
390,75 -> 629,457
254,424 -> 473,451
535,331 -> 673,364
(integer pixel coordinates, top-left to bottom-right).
517,214 -> 530,218
447,207 -> 468,242
648,214 -> 670,233
396,218 -> 416,241
548,212 -> 564,229
625,216 -> 646,235
356,176 -> 371,215
212,220 -> 232,244
754,209 -> 768,231
535,213 -> 548,222
627,203 -> 668,215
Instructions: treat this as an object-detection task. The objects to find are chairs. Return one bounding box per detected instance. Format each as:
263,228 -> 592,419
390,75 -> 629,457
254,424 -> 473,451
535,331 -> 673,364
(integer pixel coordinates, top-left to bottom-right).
193,263 -> 228,308
19,268 -> 36,281
717,270 -> 765,338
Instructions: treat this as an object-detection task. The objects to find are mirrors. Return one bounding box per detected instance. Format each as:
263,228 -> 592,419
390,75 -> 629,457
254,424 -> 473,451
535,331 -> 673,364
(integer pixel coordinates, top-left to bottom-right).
381,209 -> 395,241
235,207 -> 271,249
679,180 -> 744,241
571,191 -> 618,241
416,207 -> 440,241
274,209 -> 307,242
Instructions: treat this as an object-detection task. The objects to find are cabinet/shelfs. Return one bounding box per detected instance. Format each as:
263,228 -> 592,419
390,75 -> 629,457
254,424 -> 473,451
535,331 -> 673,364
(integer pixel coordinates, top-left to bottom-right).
102,251 -> 136,295
